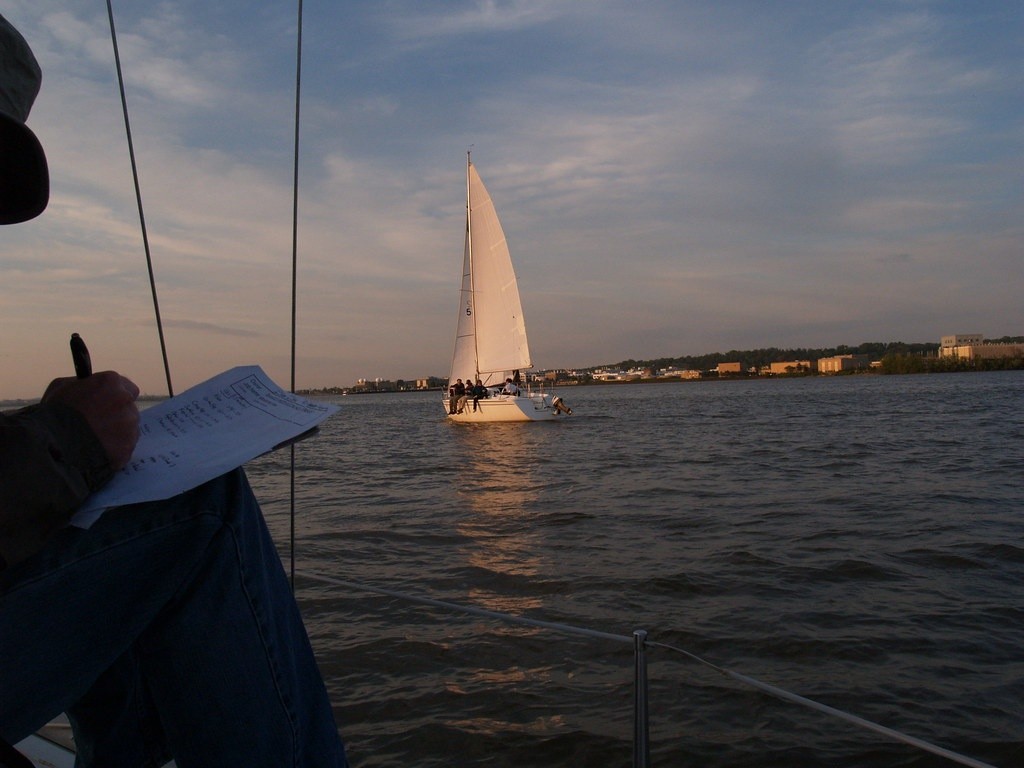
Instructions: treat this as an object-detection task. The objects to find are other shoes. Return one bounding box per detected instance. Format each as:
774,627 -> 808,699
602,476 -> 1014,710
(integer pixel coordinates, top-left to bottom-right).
457,409 -> 463,414
473,409 -> 476,412
452,410 -> 457,414
448,410 -> 453,415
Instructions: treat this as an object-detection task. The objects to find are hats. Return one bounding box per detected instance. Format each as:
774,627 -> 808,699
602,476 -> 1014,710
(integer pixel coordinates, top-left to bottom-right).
466,380 -> 470,384
0,12 -> 50,226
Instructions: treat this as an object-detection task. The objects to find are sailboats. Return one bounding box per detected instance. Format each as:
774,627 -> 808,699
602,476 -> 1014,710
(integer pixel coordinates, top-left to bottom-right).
442,151 -> 573,422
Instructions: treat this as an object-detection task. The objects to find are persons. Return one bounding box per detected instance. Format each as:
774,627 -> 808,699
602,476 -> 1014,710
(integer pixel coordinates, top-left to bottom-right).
0,16 -> 352,768
446,379 -> 465,415
501,377 -> 518,395
457,379 -> 474,414
470,380 -> 489,413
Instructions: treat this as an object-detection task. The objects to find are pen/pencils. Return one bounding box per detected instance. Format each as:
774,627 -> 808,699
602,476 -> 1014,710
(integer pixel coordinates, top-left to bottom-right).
69,329 -> 95,382
